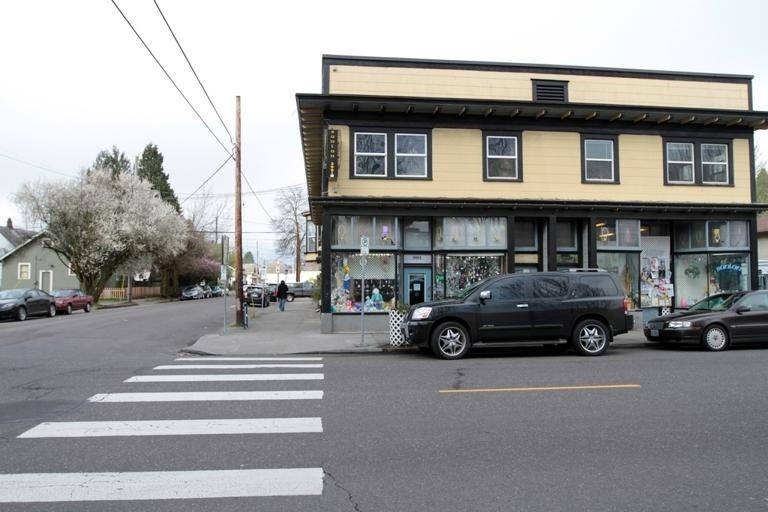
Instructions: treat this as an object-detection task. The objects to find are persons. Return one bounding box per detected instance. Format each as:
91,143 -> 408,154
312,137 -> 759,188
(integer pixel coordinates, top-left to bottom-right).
277,280 -> 290,310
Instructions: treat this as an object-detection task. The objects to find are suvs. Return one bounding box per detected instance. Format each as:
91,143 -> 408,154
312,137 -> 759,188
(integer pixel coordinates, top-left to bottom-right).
400,266 -> 635,360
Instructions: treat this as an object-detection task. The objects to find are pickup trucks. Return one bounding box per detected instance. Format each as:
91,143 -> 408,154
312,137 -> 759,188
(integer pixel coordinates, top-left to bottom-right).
283,280 -> 318,302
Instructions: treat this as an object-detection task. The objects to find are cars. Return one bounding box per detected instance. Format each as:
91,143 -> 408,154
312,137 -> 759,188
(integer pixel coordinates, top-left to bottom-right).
0,286 -> 57,322
46,289 -> 95,316
242,282 -> 280,309
644,289 -> 768,351
182,283 -> 230,300
